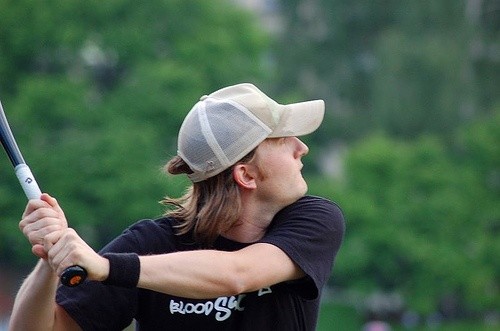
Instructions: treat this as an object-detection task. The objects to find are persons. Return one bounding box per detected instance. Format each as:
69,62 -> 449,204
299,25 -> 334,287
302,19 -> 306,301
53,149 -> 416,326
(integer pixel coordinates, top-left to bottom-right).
8,82 -> 346,331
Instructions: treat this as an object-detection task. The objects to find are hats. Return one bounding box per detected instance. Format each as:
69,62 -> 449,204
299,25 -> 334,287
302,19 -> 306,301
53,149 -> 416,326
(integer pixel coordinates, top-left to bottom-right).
177,82 -> 326,183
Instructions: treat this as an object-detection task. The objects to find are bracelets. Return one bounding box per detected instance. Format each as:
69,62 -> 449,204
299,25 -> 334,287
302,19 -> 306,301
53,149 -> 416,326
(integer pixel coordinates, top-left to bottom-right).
101,249 -> 142,290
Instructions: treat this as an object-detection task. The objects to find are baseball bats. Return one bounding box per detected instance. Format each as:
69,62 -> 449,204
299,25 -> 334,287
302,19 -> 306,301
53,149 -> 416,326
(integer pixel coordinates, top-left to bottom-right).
0,101 -> 87,287
